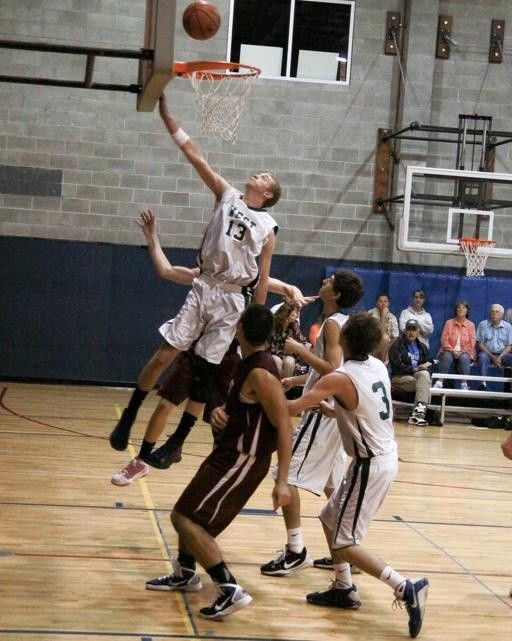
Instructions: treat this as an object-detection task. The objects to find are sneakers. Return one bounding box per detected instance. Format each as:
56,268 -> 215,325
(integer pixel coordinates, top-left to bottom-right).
144,442 -> 183,470
146,555 -> 204,593
200,583 -> 254,620
261,544 -> 314,576
111,408 -> 138,452
307,576 -> 362,610
314,555 -> 362,574
111,457 -> 151,487
408,379 -> 511,427
392,576 -> 430,639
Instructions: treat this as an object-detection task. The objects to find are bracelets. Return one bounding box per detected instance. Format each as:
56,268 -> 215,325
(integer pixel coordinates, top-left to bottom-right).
169,126 -> 191,150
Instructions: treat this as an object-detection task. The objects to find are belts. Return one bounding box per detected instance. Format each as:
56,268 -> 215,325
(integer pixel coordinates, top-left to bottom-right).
493,353 -> 501,356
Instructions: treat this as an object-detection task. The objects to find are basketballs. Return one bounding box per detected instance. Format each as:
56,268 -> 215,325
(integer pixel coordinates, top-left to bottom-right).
181,1 -> 221,40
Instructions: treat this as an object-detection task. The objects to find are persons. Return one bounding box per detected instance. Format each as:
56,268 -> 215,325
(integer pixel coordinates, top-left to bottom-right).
260,267 -> 368,577
476,304 -> 512,390
109,223 -> 318,625
366,289 -> 434,426
107,88 -> 282,470
500,433 -> 512,462
287,311 -> 431,639
432,299 -> 476,391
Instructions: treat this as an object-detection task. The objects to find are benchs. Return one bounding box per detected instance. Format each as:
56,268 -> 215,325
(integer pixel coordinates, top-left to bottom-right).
383,359 -> 512,424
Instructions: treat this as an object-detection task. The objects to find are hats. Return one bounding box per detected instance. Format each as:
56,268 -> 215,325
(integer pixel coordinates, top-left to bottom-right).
406,319 -> 418,328
